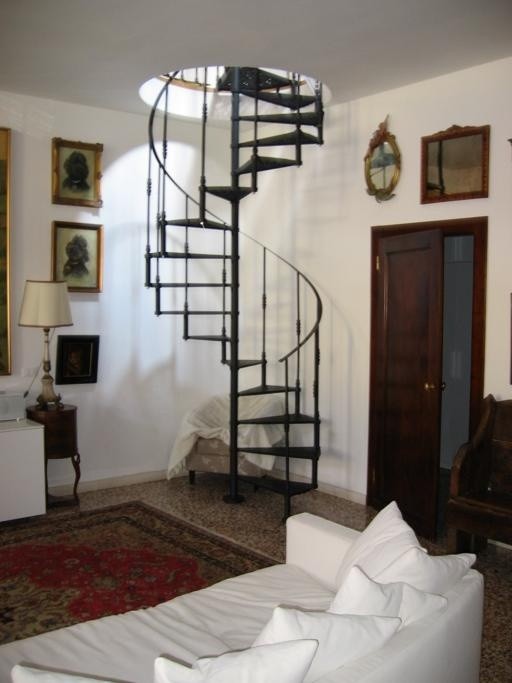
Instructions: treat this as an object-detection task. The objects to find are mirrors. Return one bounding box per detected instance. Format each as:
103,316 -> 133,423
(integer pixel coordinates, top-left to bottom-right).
420,123 -> 490,204
363,113 -> 402,203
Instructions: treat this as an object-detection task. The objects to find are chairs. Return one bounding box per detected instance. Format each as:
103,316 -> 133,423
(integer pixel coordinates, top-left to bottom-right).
445,393 -> 512,553
184,392 -> 283,491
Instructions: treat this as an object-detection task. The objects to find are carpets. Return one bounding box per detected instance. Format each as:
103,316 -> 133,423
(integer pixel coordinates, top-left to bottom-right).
0,498 -> 285,644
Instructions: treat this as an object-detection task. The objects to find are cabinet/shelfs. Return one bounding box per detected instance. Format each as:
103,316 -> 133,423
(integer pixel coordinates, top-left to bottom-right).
0,417 -> 47,525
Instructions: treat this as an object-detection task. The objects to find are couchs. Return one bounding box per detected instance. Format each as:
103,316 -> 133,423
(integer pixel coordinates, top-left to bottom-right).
0,512 -> 486,682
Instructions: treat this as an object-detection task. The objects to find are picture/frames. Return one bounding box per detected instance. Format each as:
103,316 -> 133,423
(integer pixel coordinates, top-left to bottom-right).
55,334 -> 99,384
49,134 -> 105,294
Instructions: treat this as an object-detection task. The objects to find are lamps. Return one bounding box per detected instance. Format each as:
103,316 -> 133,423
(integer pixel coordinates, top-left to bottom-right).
16,278 -> 73,409
